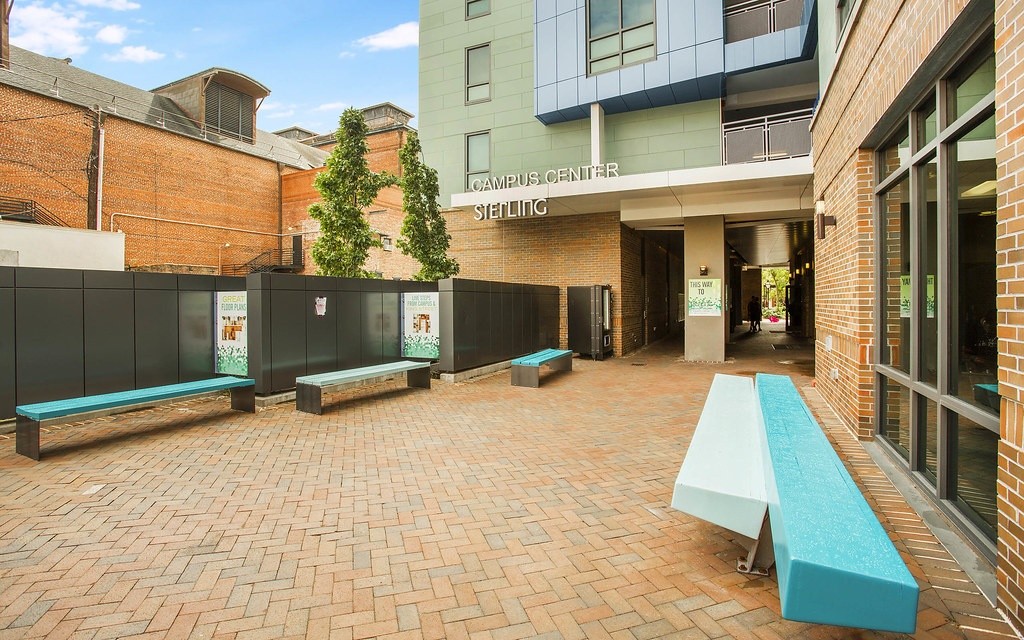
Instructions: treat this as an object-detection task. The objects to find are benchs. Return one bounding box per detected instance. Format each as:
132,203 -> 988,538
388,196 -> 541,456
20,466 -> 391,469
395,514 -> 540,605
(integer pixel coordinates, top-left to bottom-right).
14,375 -> 256,461
510,348 -> 573,388
751,372 -> 918,634
293,357 -> 433,415
671,372 -> 770,570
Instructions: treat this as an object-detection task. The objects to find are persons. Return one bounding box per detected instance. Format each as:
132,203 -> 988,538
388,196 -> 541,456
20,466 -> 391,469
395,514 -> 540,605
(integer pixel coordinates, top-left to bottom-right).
747,296 -> 757,332
755,296 -> 763,331
